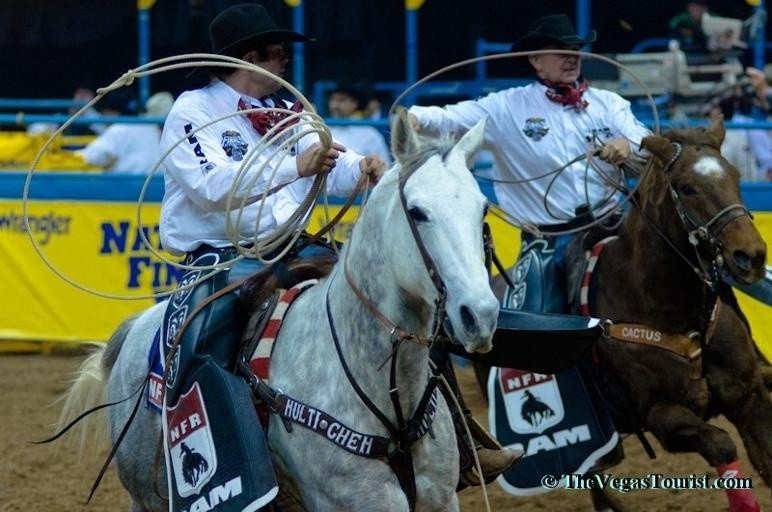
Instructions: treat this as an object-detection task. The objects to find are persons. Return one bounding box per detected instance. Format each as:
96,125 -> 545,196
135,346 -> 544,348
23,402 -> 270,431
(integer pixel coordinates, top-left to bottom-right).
409,13 -> 657,266
159,3 -> 527,477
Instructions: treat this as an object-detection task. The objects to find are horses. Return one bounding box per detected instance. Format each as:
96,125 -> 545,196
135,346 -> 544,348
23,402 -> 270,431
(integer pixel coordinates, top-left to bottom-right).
40,101 -> 502,512
471,106 -> 772,512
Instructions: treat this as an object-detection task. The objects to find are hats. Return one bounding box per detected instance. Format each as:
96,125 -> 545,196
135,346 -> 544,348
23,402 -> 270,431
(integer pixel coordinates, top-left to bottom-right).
187,4 -> 316,75
326,83 -> 367,110
511,14 -> 597,49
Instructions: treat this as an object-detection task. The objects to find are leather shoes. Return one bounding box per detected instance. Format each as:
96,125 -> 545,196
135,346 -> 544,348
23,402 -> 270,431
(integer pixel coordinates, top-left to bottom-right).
478,448 -> 513,477
